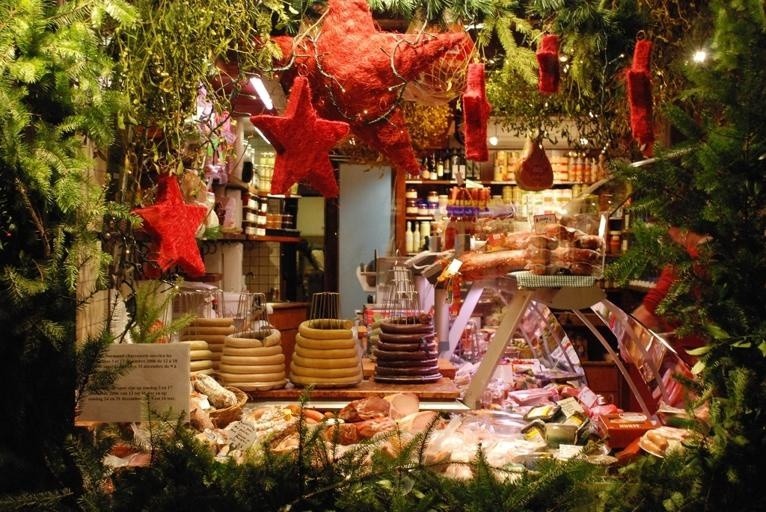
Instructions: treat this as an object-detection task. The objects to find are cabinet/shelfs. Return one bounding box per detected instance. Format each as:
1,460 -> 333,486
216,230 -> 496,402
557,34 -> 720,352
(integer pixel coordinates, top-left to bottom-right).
211,114 -> 303,245
396,150 -> 630,270
268,308 -> 307,373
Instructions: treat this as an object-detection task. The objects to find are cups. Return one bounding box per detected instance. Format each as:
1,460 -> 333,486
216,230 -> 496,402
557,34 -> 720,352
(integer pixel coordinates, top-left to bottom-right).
264,213 -> 293,228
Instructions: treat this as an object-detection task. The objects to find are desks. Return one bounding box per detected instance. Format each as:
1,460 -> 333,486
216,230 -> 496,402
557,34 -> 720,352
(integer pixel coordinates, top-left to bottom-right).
442,276 -> 613,404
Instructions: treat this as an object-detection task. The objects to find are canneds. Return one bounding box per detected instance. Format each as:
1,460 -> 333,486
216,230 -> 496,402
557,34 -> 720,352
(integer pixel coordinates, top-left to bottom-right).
427,191 -> 448,210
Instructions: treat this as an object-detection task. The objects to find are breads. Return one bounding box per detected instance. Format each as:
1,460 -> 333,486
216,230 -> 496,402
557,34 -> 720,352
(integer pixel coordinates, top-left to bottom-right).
526,224 -> 601,281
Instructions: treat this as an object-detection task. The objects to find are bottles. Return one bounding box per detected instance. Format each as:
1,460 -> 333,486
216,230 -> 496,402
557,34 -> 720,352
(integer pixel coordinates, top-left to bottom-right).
240,142 -> 276,236
405,146 -> 623,254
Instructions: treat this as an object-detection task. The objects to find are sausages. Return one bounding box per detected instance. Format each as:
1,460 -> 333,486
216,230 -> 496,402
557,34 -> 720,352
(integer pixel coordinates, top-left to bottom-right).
644,431 -> 668,457
